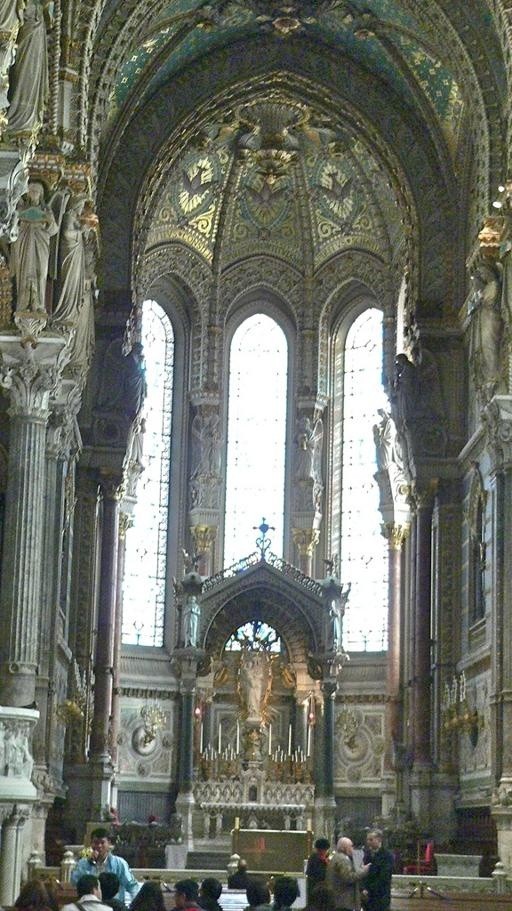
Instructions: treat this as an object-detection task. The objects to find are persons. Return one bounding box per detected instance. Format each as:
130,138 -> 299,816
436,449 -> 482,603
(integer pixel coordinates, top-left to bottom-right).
228,859 -> 250,889
244,882 -> 273,911
467,259 -> 503,399
171,879 -> 209,911
59,875 -> 114,911
52,195 -> 88,320
14,880 -> 53,911
98,872 -> 129,911
129,880 -> 166,911
306,830 -> 395,911
197,878 -> 223,911
271,877 -> 297,911
12,182 -> 60,315
71,828 -> 140,902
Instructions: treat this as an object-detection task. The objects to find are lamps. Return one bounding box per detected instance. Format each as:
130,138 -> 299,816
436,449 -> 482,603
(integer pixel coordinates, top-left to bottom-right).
57,671 -> 88,722
442,672 -> 485,735
139,701 -> 167,746
238,136 -> 300,185
336,706 -> 361,749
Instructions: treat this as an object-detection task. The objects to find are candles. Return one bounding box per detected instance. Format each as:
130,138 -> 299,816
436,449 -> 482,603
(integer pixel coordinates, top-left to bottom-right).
198,723 -> 307,766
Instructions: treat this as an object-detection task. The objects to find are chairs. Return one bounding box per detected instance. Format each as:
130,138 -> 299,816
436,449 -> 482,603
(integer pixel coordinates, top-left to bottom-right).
403,841 -> 434,876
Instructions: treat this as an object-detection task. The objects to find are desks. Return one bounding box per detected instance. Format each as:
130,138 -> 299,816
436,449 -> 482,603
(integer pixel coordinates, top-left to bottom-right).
199,801 -> 306,840
